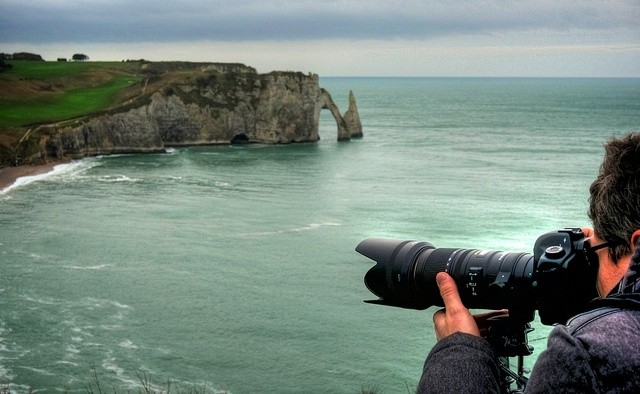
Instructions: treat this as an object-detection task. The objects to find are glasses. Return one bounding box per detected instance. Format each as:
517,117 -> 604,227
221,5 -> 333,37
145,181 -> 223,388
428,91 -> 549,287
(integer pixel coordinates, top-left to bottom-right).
583,233 -> 626,267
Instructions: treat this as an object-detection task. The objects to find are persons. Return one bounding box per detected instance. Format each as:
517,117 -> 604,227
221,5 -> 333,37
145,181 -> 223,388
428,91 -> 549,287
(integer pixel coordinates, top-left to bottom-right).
415,132 -> 640,394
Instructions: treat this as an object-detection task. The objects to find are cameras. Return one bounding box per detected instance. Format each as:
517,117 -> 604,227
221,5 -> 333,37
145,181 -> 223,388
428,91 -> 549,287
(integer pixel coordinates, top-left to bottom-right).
355,225 -> 598,357
